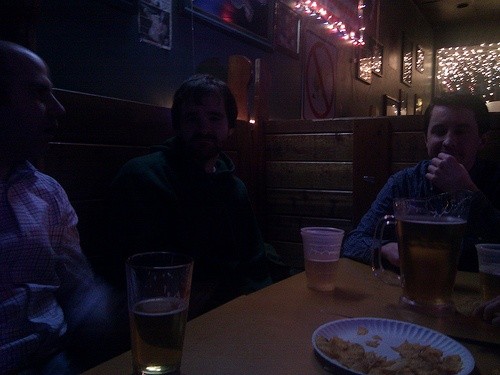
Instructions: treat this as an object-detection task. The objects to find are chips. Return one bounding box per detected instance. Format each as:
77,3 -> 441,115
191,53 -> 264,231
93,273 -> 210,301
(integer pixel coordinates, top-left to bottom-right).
314,325 -> 463,375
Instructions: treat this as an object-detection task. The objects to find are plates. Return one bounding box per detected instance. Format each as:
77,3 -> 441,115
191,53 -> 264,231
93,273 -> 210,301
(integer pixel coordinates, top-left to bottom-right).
311,317 -> 476,375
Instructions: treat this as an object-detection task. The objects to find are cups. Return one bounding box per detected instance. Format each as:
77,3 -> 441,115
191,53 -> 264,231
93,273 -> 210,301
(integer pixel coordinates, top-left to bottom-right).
476,243 -> 500,302
125,251 -> 194,375
371,196 -> 468,316
300,226 -> 344,293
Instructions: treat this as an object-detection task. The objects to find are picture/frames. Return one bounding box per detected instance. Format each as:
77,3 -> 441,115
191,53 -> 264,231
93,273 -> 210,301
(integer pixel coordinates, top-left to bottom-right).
356,31 -> 426,116
179,0 -> 303,60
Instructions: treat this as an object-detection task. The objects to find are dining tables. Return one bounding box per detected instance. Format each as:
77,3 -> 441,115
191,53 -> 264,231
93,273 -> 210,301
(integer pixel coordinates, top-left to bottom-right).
84,258 -> 500,375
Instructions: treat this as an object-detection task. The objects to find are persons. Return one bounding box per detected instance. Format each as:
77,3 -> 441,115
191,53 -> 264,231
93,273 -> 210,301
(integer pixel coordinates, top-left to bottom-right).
0,37 -> 281,375
338,93 -> 500,272
